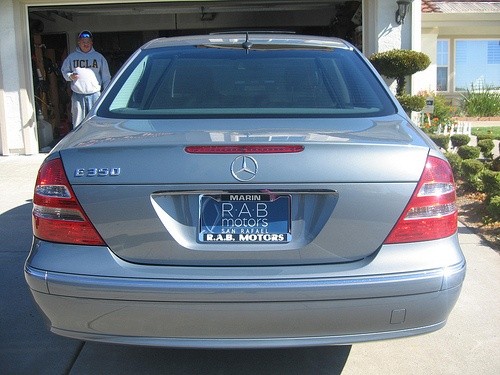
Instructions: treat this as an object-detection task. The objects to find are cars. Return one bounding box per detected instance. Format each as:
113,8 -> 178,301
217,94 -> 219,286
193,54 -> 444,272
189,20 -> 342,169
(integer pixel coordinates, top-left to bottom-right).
21,27 -> 467,349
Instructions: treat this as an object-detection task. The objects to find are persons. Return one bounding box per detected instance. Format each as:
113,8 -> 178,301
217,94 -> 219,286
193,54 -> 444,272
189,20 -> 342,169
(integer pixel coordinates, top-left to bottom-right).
60,29 -> 112,131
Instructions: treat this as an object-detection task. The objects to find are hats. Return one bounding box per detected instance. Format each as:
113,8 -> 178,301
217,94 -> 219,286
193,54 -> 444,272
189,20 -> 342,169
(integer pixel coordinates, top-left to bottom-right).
78,30 -> 92,39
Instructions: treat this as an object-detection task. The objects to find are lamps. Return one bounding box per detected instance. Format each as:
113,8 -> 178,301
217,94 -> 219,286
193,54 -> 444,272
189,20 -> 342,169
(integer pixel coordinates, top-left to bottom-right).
395,0 -> 414,25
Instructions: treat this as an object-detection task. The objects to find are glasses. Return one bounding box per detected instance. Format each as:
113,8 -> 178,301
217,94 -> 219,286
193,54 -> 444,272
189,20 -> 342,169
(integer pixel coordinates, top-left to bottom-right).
79,41 -> 93,45
